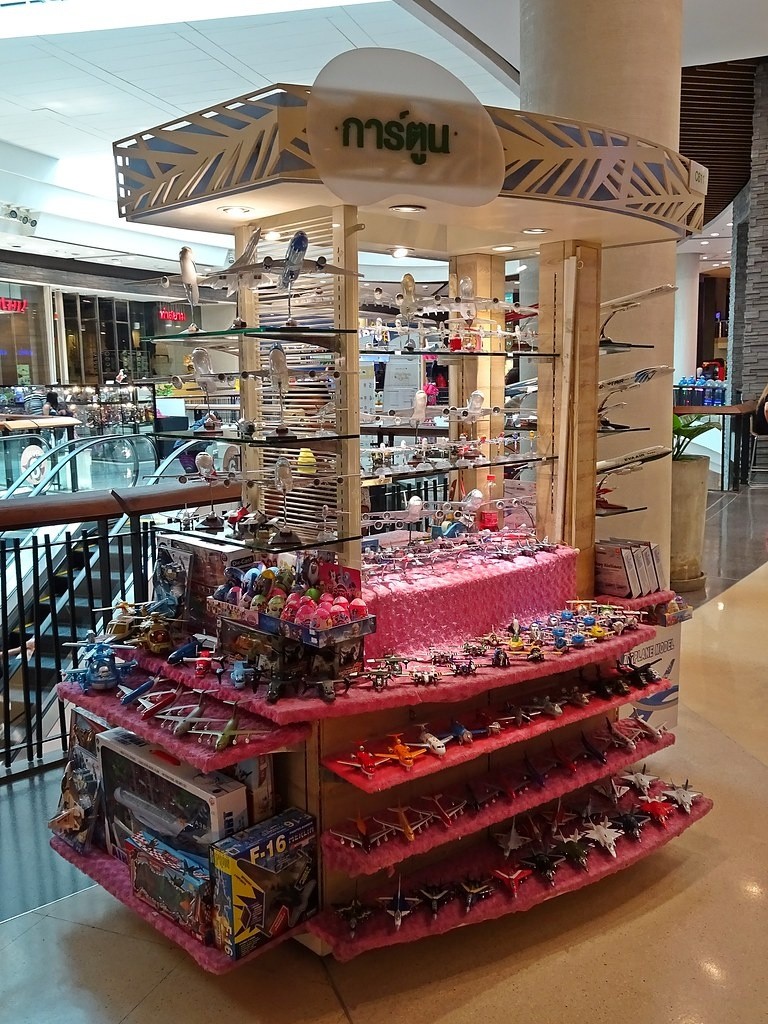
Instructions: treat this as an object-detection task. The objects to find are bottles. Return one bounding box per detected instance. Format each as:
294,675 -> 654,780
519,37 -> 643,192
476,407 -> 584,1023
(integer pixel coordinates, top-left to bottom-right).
480,474 -> 498,530
678,376 -> 726,406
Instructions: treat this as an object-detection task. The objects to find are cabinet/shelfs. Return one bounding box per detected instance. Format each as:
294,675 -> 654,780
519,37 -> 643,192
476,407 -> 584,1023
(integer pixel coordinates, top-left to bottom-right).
1,382 -> 156,463
49,612 -> 310,976
307,604 -> 714,965
147,206 -> 672,647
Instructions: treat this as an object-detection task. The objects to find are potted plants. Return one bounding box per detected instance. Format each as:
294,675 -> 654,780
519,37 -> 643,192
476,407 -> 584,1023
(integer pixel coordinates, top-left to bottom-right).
670,413 -> 724,593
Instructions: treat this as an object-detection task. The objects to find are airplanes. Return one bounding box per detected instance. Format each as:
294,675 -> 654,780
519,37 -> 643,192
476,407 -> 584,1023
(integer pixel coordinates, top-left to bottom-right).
124,225 -> 676,584
91,595 -> 703,940
116,666 -> 171,709
154,688 -> 230,736
187,699 -> 271,752
137,672 -> 192,721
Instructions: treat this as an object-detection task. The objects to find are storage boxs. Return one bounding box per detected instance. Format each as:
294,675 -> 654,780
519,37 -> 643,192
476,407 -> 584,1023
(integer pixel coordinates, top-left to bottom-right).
46,704 -> 323,962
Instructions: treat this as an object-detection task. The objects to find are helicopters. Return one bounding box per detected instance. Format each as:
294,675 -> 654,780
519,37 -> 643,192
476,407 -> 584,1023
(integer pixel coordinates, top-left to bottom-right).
61,635 -> 137,695
